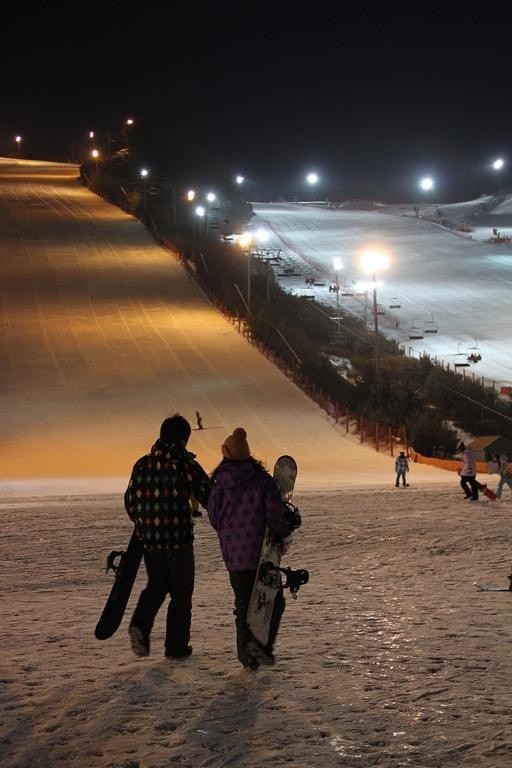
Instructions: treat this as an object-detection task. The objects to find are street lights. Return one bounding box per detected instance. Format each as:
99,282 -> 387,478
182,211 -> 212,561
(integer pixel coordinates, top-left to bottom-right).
356,237 -> 387,395
332,255 -> 347,334
88,117 -> 217,261
491,157 -> 504,193
16,134 -> 23,157
238,227 -> 254,311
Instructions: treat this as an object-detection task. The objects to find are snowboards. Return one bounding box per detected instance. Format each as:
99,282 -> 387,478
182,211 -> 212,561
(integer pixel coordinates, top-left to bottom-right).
457,468 -> 497,501
244,455 -> 298,646
95,529 -> 145,641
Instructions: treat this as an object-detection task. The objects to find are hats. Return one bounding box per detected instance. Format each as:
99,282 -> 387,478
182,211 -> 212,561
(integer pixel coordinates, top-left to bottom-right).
221,428 -> 252,461
457,441 -> 464,449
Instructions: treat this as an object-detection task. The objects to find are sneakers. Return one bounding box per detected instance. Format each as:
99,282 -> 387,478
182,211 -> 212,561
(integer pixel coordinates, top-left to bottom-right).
237,641 -> 276,671
465,493 -> 478,500
129,621 -> 149,658
164,643 -> 192,659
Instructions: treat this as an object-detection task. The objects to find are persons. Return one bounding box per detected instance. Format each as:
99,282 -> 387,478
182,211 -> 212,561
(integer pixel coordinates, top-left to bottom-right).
452,439 -> 482,504
120,407 -> 219,662
392,450 -> 410,489
492,452 -> 512,496
209,424 -> 302,675
